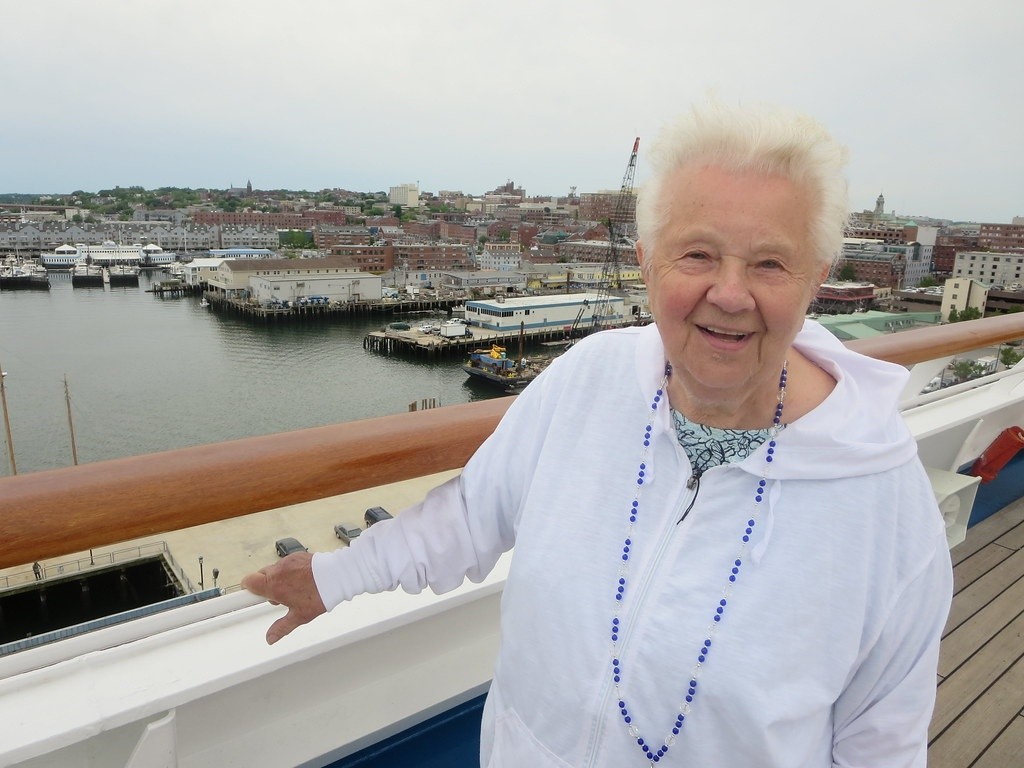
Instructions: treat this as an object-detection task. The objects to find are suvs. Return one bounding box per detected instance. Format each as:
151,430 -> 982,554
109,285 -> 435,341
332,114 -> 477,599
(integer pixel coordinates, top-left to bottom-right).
364,506 -> 394,528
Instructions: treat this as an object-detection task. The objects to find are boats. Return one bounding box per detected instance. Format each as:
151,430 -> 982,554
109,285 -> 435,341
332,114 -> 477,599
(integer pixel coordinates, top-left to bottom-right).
0,241 -> 178,286
464,320 -> 543,390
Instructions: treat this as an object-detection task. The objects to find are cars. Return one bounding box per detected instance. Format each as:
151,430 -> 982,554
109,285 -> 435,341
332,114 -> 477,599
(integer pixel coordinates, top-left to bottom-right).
275,536 -> 308,557
334,521 -> 363,546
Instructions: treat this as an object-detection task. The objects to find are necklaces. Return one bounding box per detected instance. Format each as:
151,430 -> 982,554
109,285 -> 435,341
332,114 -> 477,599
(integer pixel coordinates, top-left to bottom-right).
611,359 -> 790,768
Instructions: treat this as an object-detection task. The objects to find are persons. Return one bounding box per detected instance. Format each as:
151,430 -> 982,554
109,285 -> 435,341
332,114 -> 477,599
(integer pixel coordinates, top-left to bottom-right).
243,104 -> 954,768
